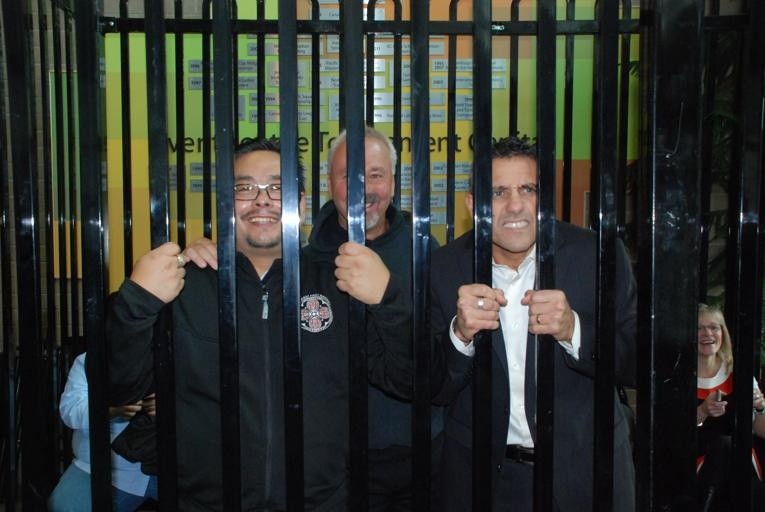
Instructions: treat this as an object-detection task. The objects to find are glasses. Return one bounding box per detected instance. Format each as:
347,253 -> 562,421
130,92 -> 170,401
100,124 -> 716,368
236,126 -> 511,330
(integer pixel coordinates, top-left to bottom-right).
232,179 -> 286,204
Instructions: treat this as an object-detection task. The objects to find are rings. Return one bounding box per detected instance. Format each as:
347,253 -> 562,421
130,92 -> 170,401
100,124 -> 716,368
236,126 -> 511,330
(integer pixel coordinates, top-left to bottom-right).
536,314 -> 541,324
177,254 -> 185,267
477,299 -> 484,308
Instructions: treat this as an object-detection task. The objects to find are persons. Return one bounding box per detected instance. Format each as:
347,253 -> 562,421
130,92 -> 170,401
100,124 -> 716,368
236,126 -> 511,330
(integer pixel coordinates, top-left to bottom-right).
302,125 -> 442,512
408,135 -> 636,511
82,134 -> 449,511
48,352 -> 157,512
696,301 -> 765,512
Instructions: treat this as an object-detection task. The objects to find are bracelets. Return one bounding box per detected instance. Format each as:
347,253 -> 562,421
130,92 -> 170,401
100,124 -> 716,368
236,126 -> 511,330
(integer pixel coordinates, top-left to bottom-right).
755,409 -> 765,415
